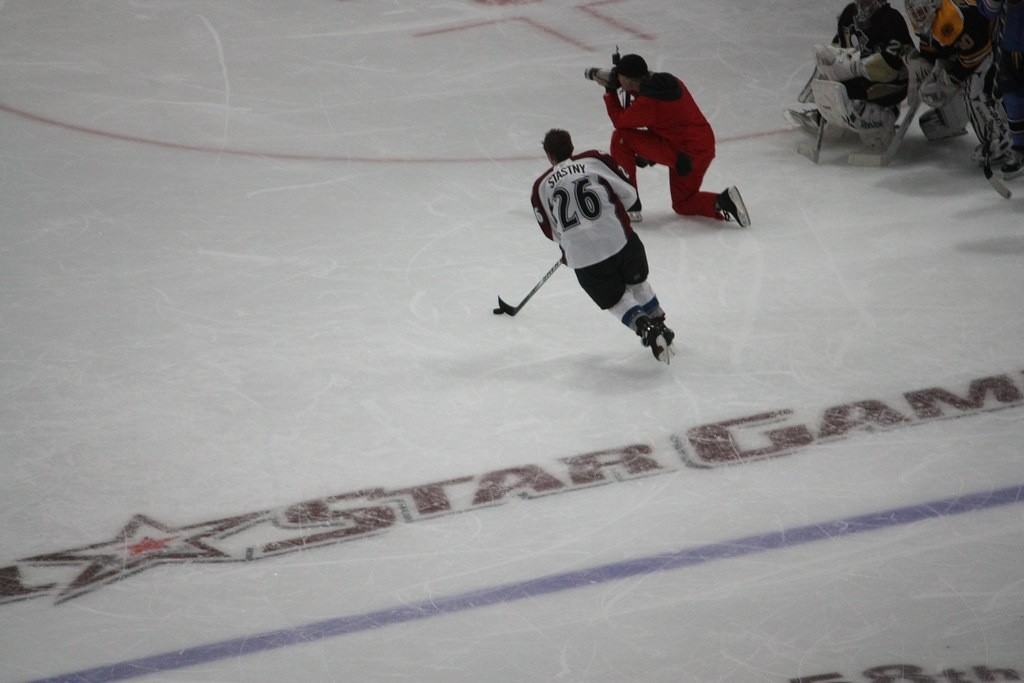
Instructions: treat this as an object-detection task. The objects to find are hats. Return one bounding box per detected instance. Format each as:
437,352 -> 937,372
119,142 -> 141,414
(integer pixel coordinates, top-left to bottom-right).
618,54 -> 647,76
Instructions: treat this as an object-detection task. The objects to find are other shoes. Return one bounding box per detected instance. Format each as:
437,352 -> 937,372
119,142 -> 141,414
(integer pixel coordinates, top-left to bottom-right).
804,109 -> 827,127
884,105 -> 900,126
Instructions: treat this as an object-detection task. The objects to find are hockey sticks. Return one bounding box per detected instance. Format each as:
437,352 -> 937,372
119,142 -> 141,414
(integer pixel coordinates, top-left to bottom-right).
494,255 -> 568,319
842,54 -> 950,172
793,112 -> 831,169
977,90 -> 1017,205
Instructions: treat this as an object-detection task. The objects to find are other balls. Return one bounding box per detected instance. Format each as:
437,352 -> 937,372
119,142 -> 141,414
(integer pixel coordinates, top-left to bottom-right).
491,306 -> 506,316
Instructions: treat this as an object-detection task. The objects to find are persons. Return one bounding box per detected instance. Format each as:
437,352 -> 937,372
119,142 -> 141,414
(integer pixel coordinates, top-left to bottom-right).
594,54 -> 750,228
782,0 -> 907,150
905,0 -> 1024,179
530,129 -> 673,363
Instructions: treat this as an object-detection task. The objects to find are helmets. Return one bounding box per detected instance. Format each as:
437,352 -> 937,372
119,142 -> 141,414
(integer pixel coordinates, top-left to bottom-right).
855,0 -> 883,22
903,0 -> 942,35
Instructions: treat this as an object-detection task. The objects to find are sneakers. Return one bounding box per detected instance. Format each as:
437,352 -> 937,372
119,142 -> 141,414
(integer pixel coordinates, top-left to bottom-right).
627,194 -> 642,222
978,146 -> 1024,180
635,315 -> 675,365
717,185 -> 751,228
